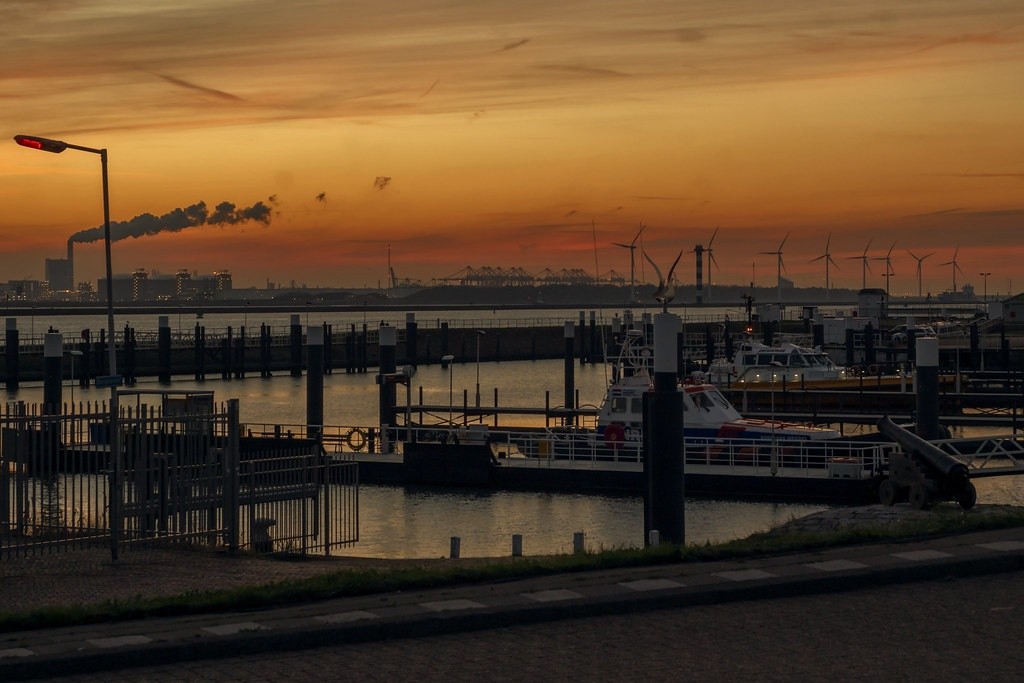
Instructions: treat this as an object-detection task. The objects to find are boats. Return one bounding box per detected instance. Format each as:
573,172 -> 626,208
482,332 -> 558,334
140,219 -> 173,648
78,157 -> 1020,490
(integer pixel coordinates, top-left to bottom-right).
592,220 -> 835,466
691,324 -> 969,413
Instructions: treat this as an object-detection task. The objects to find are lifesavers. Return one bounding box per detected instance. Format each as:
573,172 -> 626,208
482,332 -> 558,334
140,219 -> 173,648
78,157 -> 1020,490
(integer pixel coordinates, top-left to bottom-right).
346,429 -> 366,449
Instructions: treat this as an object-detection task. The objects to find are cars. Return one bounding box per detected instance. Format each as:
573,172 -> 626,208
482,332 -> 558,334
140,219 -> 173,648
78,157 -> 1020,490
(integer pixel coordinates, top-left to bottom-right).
888,321 -> 962,344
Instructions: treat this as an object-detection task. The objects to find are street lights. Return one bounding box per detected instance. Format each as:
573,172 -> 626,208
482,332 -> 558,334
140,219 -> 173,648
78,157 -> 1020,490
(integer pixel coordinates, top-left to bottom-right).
14,135 -> 122,532
442,355 -> 454,431
979,273 -> 991,349
476,331 -> 488,408
